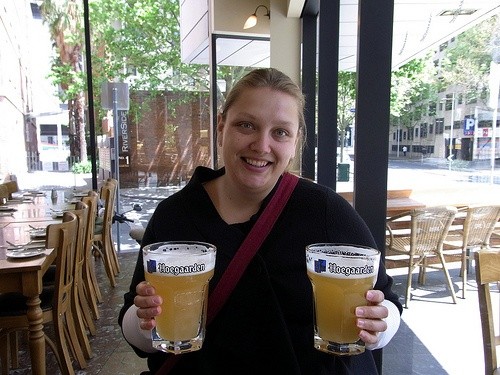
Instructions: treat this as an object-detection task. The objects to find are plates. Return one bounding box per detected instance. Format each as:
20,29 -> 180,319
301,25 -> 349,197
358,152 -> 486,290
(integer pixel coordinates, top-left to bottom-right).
51,213 -> 63,217
28,228 -> 46,236
7,247 -> 45,258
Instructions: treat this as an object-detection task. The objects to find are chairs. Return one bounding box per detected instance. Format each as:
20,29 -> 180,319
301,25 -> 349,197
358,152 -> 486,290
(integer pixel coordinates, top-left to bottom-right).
386,205 -> 500,375
0,178 -> 121,375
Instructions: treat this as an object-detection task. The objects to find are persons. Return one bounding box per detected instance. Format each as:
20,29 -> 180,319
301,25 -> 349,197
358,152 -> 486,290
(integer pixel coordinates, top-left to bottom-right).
118,68 -> 404,374
402,145 -> 407,156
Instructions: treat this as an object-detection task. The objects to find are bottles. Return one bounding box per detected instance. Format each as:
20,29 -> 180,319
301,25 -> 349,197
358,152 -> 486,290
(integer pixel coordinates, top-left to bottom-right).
51,187 -> 57,199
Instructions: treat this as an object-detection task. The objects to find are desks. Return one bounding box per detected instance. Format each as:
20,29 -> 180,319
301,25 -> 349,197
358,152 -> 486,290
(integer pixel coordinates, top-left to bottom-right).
351,188 -> 500,234
0,189 -> 98,223
0,220 -> 63,375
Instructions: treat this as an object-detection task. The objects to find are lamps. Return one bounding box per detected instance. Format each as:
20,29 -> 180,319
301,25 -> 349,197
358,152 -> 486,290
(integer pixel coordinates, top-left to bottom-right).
243,5 -> 270,30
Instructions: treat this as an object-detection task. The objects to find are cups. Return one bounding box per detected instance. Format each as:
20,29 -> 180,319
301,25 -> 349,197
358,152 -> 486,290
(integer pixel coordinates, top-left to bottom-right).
305,242 -> 380,355
143,241 -> 216,354
